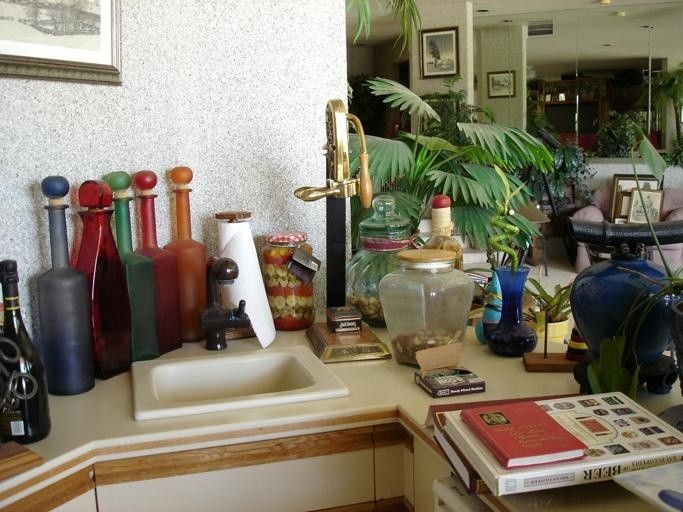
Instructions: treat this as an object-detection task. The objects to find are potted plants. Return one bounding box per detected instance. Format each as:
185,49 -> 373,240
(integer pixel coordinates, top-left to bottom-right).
587,123 -> 682,397
516,144 -> 590,239
516,274 -> 575,345
483,162 -> 538,356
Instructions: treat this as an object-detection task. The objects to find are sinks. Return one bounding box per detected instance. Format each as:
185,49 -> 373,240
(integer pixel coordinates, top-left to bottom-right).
132,346 -> 350,421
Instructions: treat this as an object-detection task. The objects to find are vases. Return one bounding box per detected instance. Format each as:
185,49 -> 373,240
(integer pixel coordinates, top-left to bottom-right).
475,266 -> 504,343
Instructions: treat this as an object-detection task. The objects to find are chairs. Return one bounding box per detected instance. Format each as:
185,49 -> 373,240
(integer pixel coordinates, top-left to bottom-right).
571,183 -> 683,278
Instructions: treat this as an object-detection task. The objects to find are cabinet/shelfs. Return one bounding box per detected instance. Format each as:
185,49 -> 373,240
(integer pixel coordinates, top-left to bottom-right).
542,78 -> 602,130
91,427 -> 374,512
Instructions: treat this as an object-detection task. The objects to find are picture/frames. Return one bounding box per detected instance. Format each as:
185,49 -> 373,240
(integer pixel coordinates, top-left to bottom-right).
420,26 -> 460,79
614,191 -> 630,224
419,94 -> 459,134
485,69 -> 517,99
608,174 -> 662,222
0,1 -> 124,87
627,188 -> 664,225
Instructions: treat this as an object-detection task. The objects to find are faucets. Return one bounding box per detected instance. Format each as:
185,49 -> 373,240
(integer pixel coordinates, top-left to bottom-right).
199,258 -> 250,350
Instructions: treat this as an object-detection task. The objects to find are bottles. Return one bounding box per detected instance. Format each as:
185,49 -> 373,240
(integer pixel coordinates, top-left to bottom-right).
34,167 -> 205,396
0,260 -> 53,442
424,195 -> 464,271
346,195 -> 416,328
378,249 -> 474,369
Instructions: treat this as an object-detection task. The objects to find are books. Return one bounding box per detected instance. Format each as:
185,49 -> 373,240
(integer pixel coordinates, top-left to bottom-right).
421,390 -> 681,511
